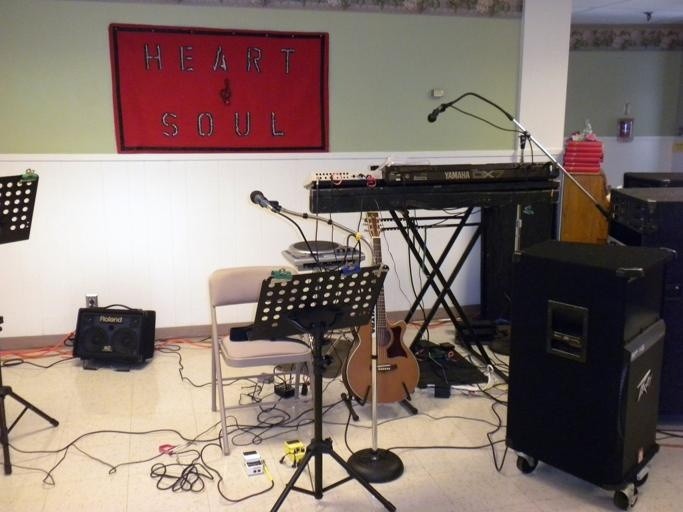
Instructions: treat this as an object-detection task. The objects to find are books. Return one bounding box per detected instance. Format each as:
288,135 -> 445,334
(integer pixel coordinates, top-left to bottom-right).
561,138 -> 608,175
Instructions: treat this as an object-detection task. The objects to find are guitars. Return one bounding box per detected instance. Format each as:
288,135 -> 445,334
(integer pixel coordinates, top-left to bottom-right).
343,211 -> 418,404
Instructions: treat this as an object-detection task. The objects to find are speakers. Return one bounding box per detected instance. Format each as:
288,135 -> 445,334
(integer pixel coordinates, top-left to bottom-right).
504,241 -> 678,491
71,307 -> 156,365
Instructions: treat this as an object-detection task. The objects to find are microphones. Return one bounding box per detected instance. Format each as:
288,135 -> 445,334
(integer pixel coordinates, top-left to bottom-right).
427,103 -> 447,122
250,191 -> 279,215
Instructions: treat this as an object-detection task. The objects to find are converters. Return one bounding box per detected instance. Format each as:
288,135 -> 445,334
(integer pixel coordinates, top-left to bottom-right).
275,383 -> 296,399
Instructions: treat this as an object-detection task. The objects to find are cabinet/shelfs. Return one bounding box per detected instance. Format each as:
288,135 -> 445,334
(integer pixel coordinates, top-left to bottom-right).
560,169 -> 608,244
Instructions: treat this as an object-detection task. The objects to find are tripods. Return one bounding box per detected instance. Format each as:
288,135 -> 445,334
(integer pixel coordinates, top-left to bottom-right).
0,316 -> 59,475
268,323 -> 397,512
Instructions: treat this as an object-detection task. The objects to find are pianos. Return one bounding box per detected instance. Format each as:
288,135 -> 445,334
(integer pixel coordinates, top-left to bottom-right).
307,164 -> 560,211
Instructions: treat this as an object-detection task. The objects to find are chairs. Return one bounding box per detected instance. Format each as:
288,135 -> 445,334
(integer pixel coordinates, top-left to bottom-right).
209,266 -> 316,456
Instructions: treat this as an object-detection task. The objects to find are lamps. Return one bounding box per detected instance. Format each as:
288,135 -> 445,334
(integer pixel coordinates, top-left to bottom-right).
617,117 -> 634,141
